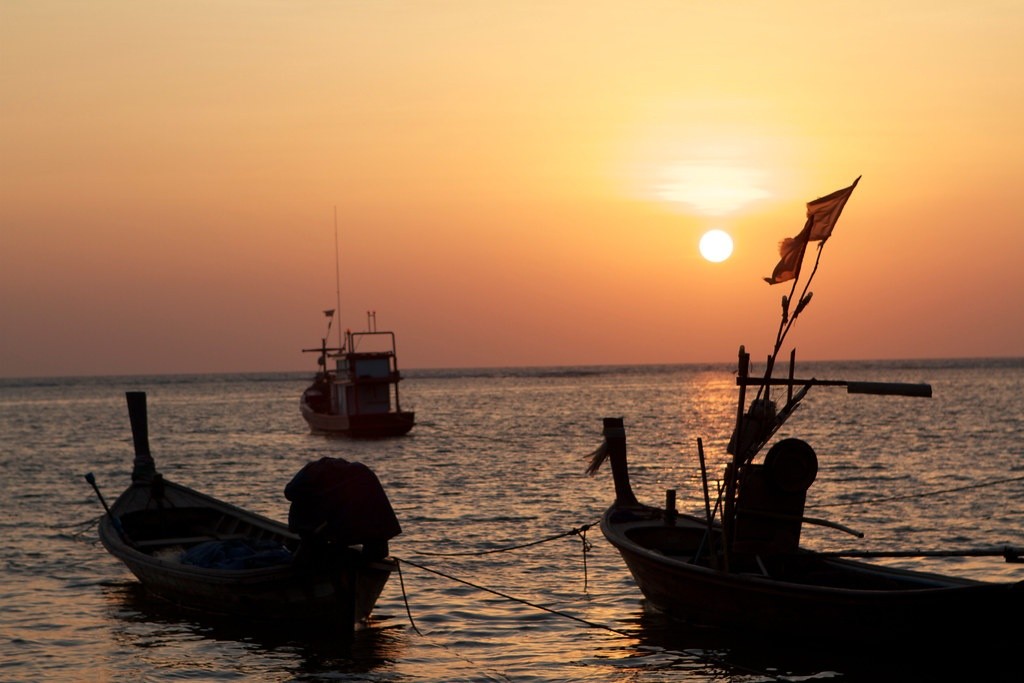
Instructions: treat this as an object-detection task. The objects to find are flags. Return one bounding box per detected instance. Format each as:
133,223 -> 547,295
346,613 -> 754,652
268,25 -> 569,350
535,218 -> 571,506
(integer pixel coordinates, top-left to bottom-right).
761,216 -> 813,284
325,308 -> 334,316
800,185 -> 853,241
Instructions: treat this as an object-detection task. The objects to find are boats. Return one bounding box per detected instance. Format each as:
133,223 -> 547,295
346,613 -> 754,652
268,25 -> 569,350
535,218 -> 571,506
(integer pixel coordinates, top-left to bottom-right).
597,345 -> 1024,683
70,390 -> 399,622
298,307 -> 417,437
